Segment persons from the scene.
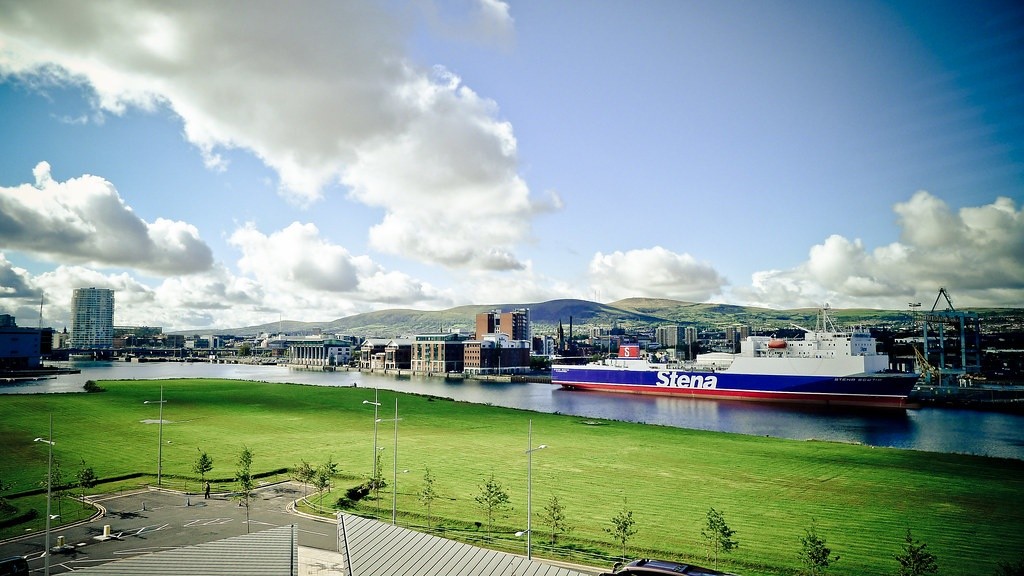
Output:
[711,362,716,373]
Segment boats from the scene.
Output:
[549,308,922,408]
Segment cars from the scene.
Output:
[596,558,737,576]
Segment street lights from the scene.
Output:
[362,400,385,490]
[375,417,409,526]
[143,400,171,485]
[514,444,549,560]
[33,437,60,576]
